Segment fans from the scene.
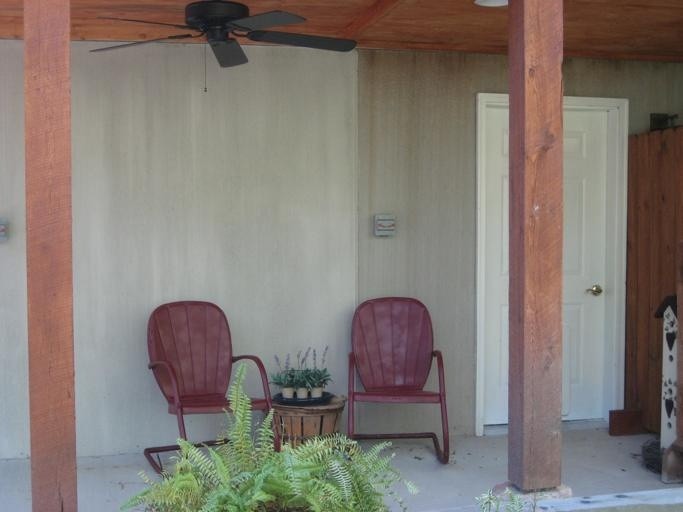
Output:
[87,0,358,70]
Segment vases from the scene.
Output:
[280,387,322,399]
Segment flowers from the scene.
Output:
[267,345,335,388]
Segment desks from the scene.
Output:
[270,392,346,464]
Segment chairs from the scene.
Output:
[141,299,282,486]
[346,295,449,466]
